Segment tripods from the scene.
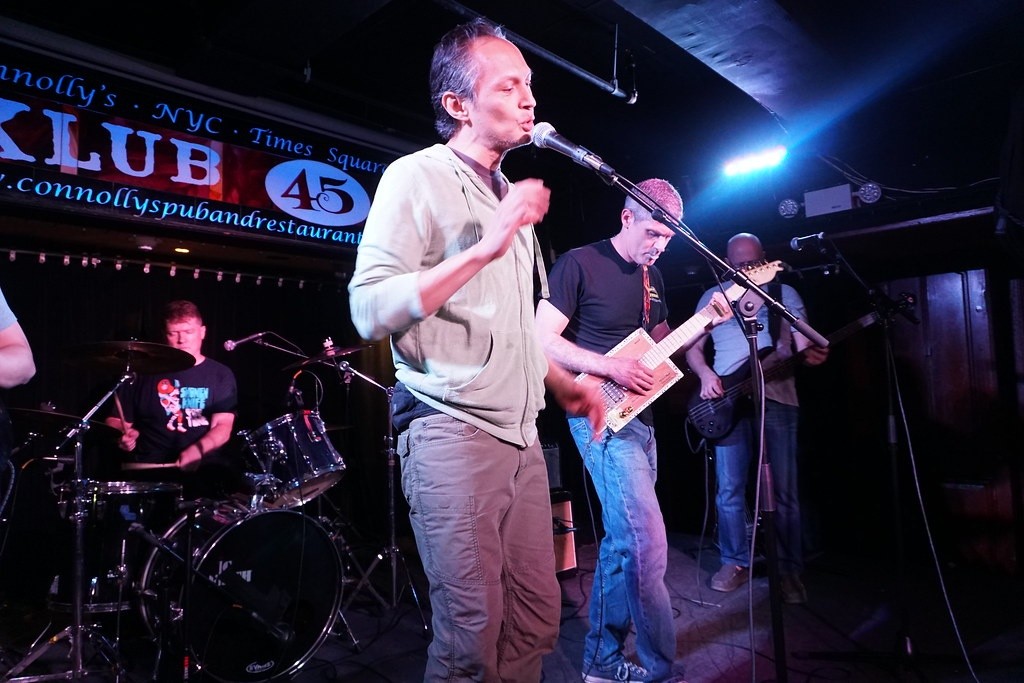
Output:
[0,358,139,683]
[318,362,432,642]
[792,245,986,683]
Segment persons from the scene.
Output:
[348,19,606,683]
[108,299,237,484]
[686,232,829,603]
[0,287,36,388]
[536,179,733,683]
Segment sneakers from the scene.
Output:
[711,564,750,592]
[583,662,648,683]
[781,578,807,604]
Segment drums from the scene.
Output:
[235,408,346,511]
[136,495,347,683]
[49,478,185,617]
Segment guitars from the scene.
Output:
[686,290,916,440]
[571,259,783,434]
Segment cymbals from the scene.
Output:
[5,404,125,443]
[73,339,198,382]
[279,343,375,375]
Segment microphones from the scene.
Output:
[284,379,296,407]
[274,621,296,647]
[532,121,616,177]
[223,332,266,351]
[552,528,576,534]
[790,232,825,250]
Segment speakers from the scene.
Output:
[551,489,579,579]
